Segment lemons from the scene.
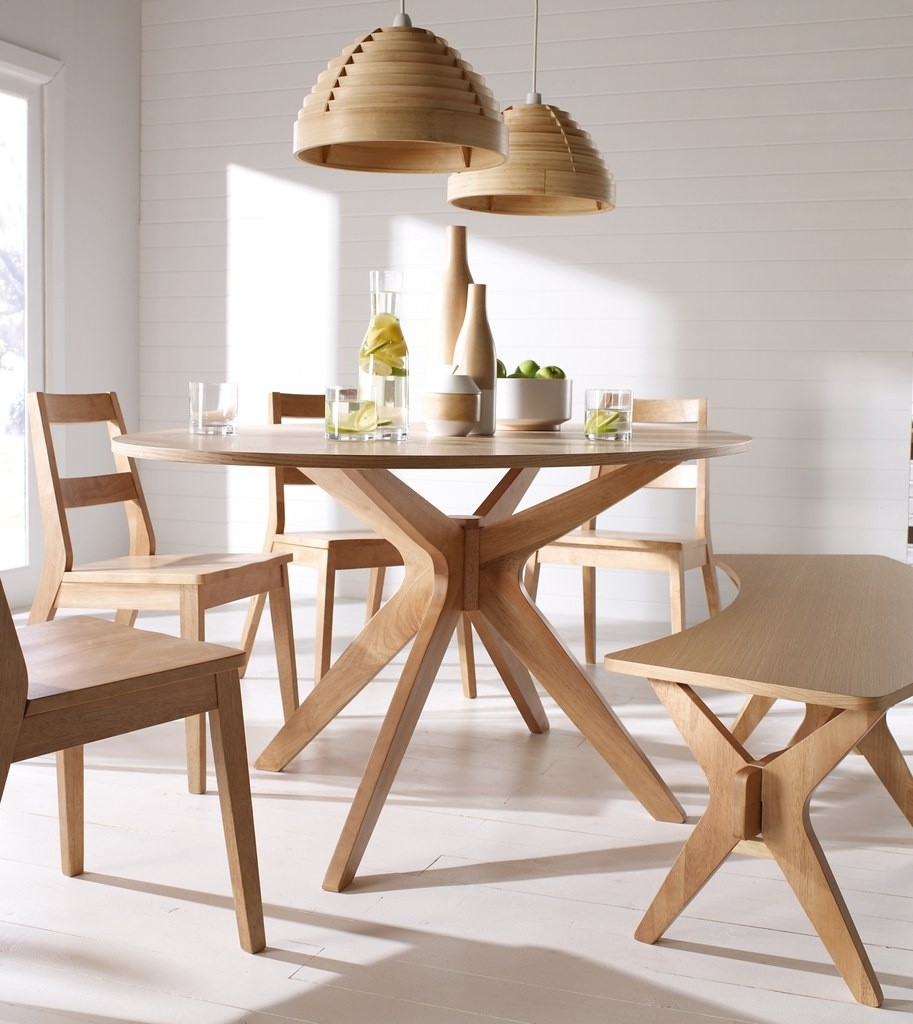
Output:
[584,409,621,433]
[326,402,391,433]
[359,312,407,377]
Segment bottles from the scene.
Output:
[359,270,410,442]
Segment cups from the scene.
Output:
[188,381,238,436]
[584,388,634,443]
[324,385,377,443]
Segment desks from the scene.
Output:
[118,424,756,895]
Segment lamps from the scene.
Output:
[447,0,617,218]
[293,0,510,173]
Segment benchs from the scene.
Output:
[606,553,913,1009]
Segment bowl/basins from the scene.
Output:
[496,377,573,431]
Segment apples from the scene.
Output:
[496,358,566,378]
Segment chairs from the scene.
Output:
[0,576,268,958]
[524,397,721,669]
[238,393,478,701]
[27,390,302,794]
